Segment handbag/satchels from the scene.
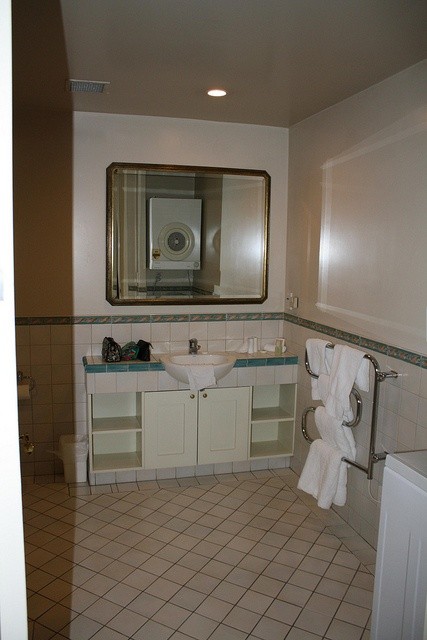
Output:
[102,337,122,362]
[122,341,140,360]
[137,339,153,361]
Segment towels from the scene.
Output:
[317,374,354,425]
[184,364,216,393]
[314,406,357,468]
[333,344,370,401]
[305,338,333,402]
[297,438,347,510]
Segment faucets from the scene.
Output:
[188,337,202,353]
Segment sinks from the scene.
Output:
[161,355,238,384]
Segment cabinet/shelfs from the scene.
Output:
[370,449,426,639]
[250,383,298,472]
[88,392,144,485]
[144,387,250,481]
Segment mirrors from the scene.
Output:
[106,162,271,306]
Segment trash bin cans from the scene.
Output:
[58,433,88,483]
